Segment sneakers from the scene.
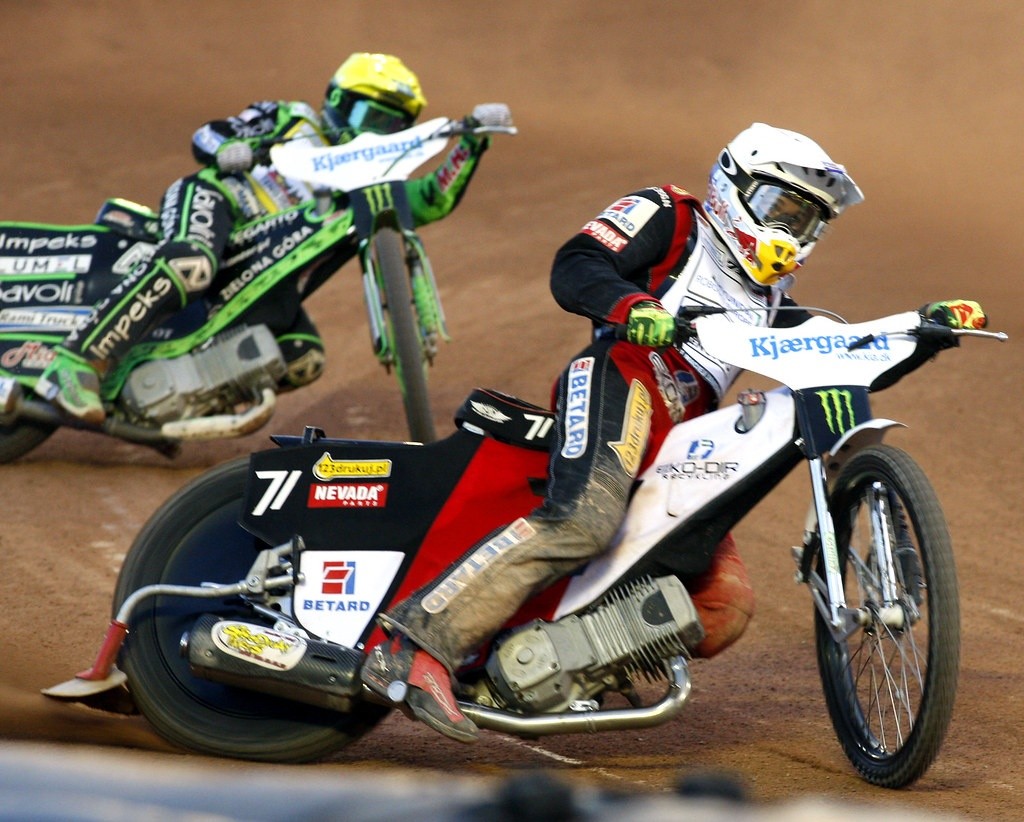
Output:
[34,353,106,425]
[361,631,481,745]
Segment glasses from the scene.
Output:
[325,84,409,135]
[717,147,831,245]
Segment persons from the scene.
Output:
[34,52,514,421]
[359,123,985,745]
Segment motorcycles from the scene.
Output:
[38,304,1012,792]
[0,102,524,469]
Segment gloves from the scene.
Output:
[626,299,679,347]
[467,102,513,138]
[918,298,986,330]
[215,137,254,175]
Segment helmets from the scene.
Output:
[702,122,865,287]
[320,52,430,146]
